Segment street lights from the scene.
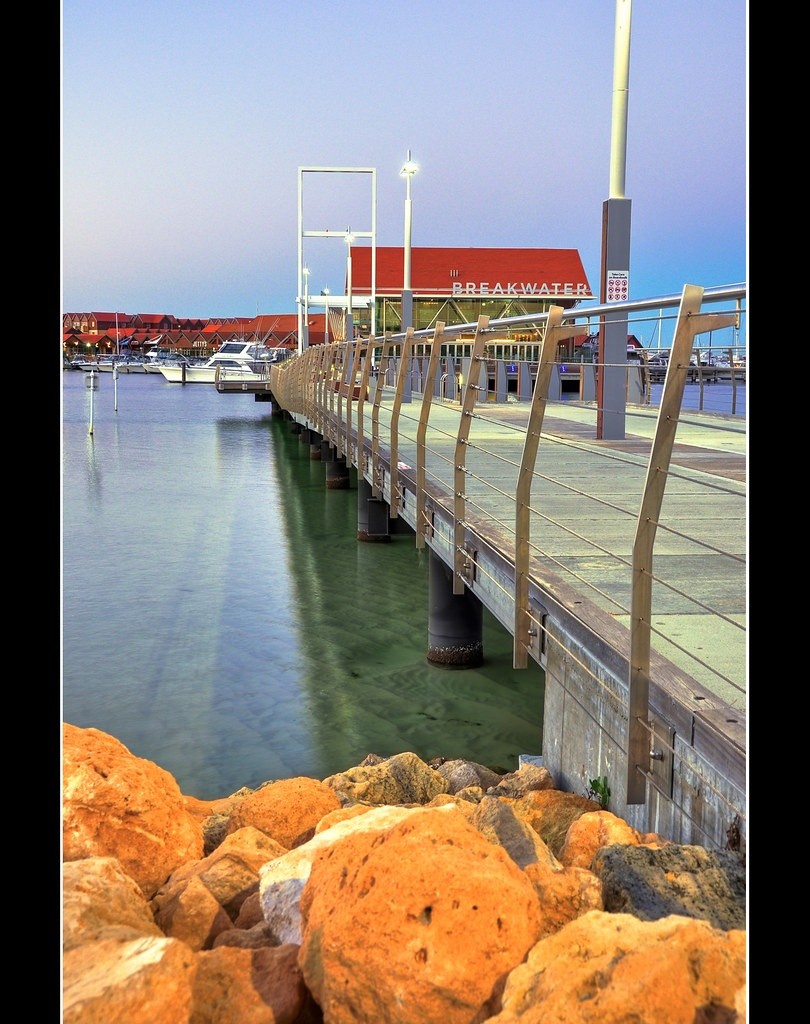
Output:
[344,225,354,384]
[324,283,330,372]
[302,262,310,351]
[399,149,419,403]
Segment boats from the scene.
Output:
[63,315,210,374]
[159,317,296,384]
[648,308,746,379]
